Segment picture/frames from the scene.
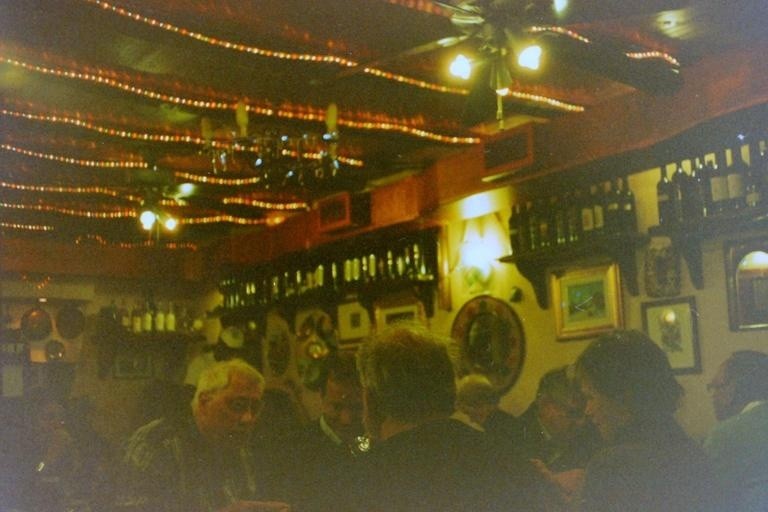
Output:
[641,295,704,377]
[113,353,153,379]
[547,259,624,343]
[335,291,427,351]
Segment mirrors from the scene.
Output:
[723,236,767,331]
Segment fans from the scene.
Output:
[326,0,687,82]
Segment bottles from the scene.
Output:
[330,243,431,287]
[654,130,768,221]
[222,272,263,313]
[507,171,636,253]
[264,253,329,300]
[97,286,207,336]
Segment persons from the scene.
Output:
[1,325,768,510]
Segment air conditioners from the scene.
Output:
[476,122,554,184]
[316,191,369,232]
